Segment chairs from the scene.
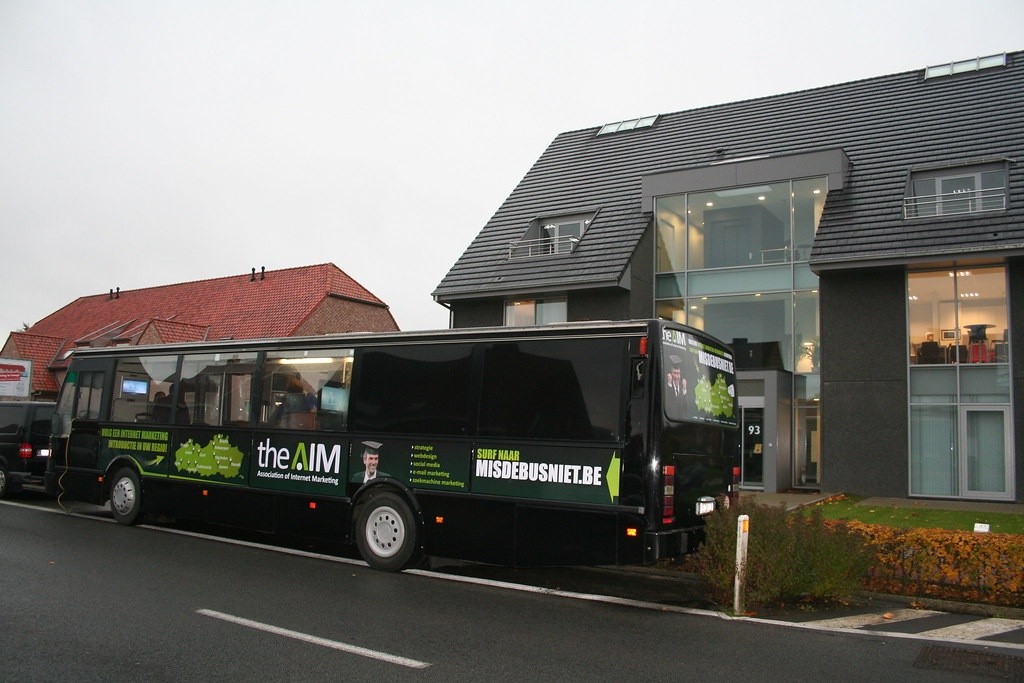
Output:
[951,344,967,363]
[277,412,317,431]
[921,342,940,364]
[989,329,1009,363]
[995,343,1008,363]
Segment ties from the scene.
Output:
[676,386,679,397]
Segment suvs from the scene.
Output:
[0,400,56,500]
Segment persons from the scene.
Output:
[666,355,690,419]
[267,383,308,425]
[146,383,191,425]
[352,440,392,483]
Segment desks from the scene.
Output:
[797,244,811,261]
[760,248,797,264]
[963,324,997,362]
[915,345,947,364]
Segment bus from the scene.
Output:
[45,317,738,573]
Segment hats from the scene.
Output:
[669,355,683,369]
[361,441,383,455]
